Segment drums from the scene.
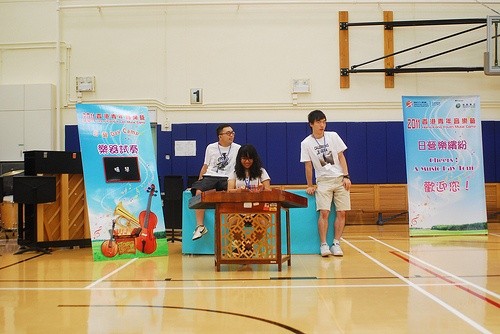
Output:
[2,194,26,230]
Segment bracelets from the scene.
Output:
[343,174,351,179]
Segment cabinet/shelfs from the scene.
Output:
[0,84,57,203]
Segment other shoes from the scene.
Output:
[232,246,252,258]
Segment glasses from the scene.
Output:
[219,131,235,136]
[241,157,254,162]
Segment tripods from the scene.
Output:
[12,175,57,255]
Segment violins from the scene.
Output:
[135,183,158,254]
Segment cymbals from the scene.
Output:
[0,169,24,178]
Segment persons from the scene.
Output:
[191,124,243,241]
[227,143,271,258]
[300,110,352,257]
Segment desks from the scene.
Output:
[182,188,336,253]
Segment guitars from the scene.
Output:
[101,219,118,257]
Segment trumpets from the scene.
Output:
[113,201,141,229]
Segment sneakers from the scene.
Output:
[330,243,343,256]
[192,224,208,240]
[320,242,332,256]
